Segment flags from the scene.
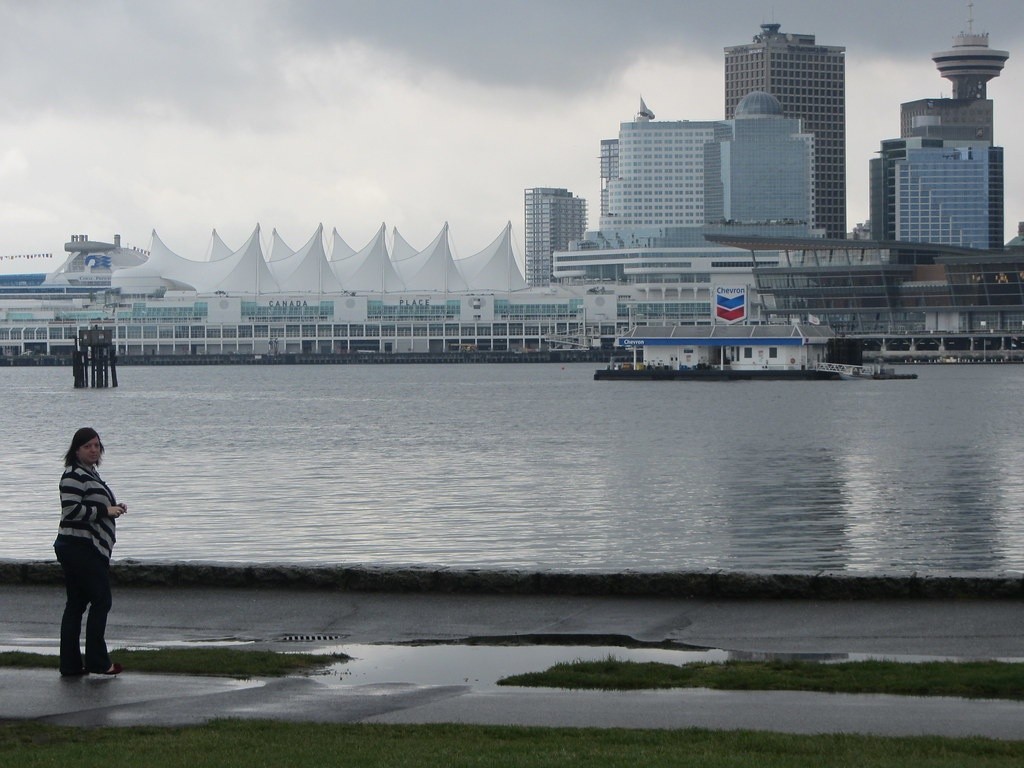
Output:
[809,315,820,325]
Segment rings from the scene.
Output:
[117,511,120,513]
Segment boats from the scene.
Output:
[0,233,195,320]
[594,325,919,380]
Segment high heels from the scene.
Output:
[58,664,88,676]
[106,662,124,675]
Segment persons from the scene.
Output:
[53,427,128,676]
